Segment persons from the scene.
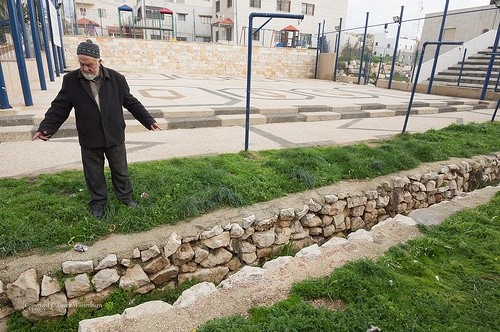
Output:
[32,39,162,220]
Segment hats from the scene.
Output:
[76,39,101,58]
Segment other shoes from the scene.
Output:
[119,195,138,210]
[90,206,108,221]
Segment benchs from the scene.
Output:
[427,46,500,91]
